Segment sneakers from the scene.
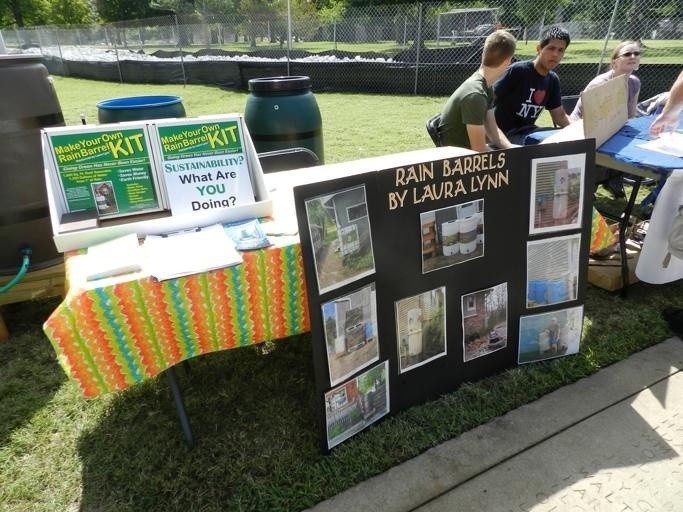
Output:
[602,182,626,198]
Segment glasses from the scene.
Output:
[613,51,640,58]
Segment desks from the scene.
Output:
[63,145,488,449]
[524,107,683,300]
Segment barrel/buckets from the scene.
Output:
[0,53,66,276]
[244,74,323,167]
[95,95,187,124]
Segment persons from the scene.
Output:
[569,37,642,135]
[649,70,683,343]
[426,30,516,155]
[494,24,570,146]
